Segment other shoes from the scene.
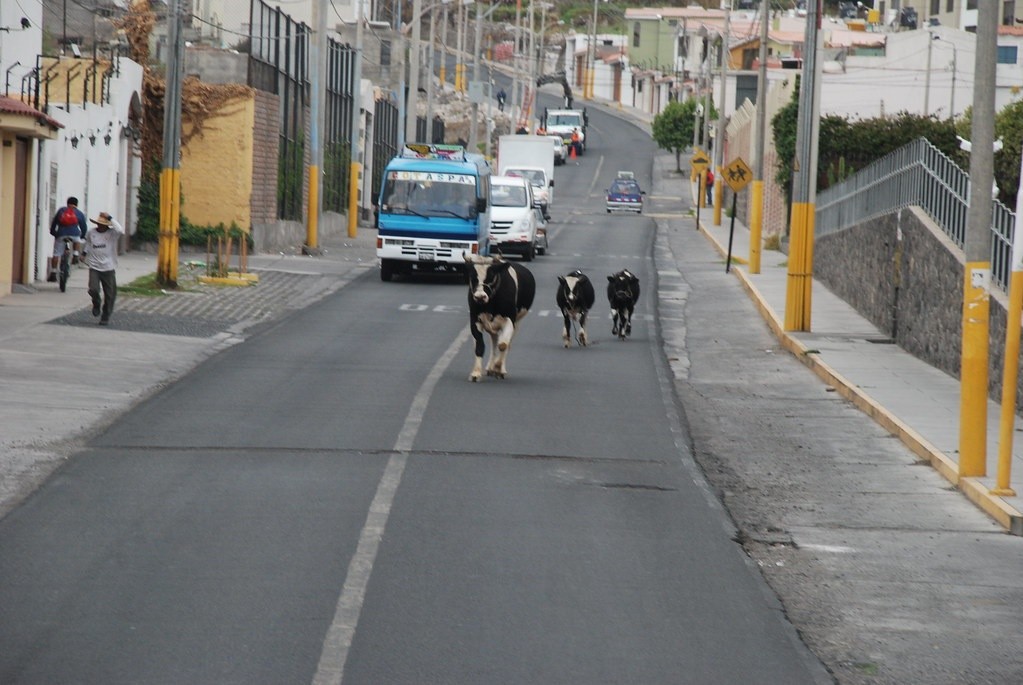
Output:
[72,255,78,264]
[99,318,110,326]
[50,272,56,282]
[92,305,101,317]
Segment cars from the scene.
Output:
[604,180,646,214]
[534,206,552,252]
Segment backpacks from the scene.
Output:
[60,206,78,226]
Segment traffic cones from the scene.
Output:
[570,147,577,159]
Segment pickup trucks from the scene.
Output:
[547,135,569,164]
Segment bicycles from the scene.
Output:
[57,238,73,291]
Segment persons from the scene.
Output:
[571,128,580,154]
[443,188,469,207]
[80,212,125,325]
[50,197,87,293]
[497,88,506,111]
[706,169,713,205]
[536,127,546,135]
[519,124,528,134]
[388,186,411,211]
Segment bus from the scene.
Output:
[376,142,493,282]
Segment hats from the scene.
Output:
[90,213,112,225]
[68,197,78,206]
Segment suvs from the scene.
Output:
[490,176,541,260]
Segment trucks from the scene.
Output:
[545,108,587,149]
[497,133,555,213]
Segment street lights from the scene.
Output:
[655,14,687,104]
[506,19,564,118]
[424,1,475,146]
[933,35,956,122]
[397,0,450,158]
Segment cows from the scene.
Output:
[461,249,535,382]
[555,269,595,349]
[606,269,641,341]
